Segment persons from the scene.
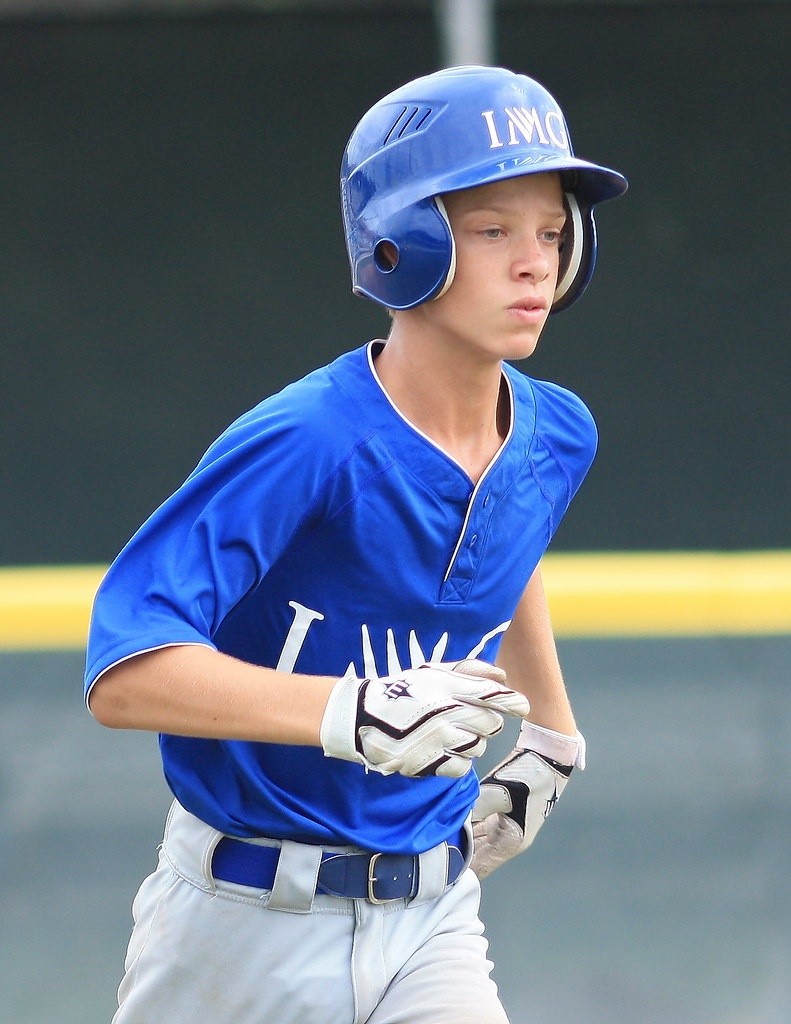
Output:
[87,66,626,1024]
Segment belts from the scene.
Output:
[212,832,470,907]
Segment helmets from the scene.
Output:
[338,62,626,312]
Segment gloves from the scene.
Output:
[321,658,529,779]
[470,722,587,879]
